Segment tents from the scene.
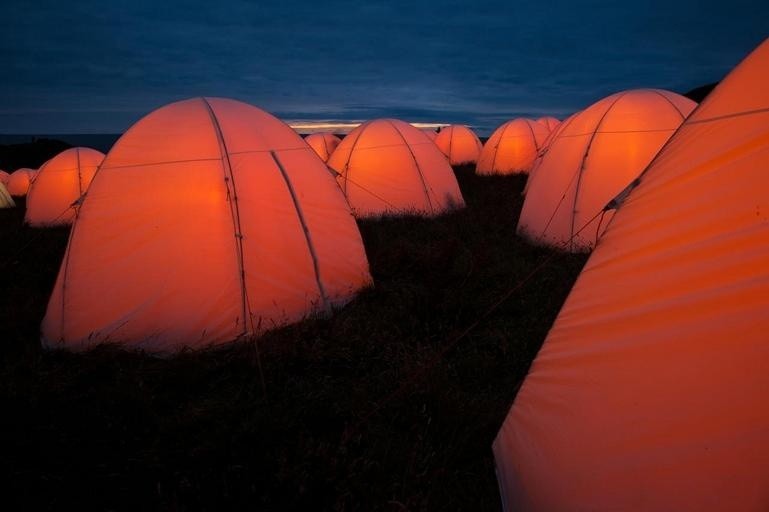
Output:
[521,111,582,195]
[0,170,10,185]
[424,129,439,141]
[6,168,39,196]
[435,124,483,166]
[0,182,16,208]
[492,36,769,512]
[39,96,375,354]
[326,118,467,220]
[304,132,342,163]
[515,87,699,254]
[535,116,561,131]
[475,118,550,176]
[25,146,107,227]
